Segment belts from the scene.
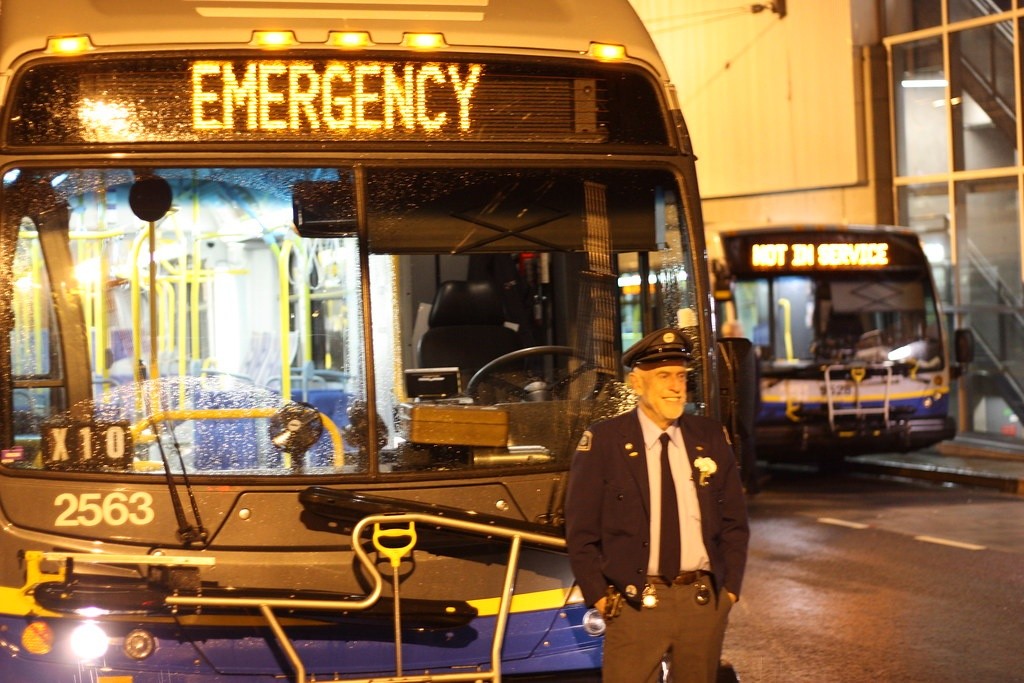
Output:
[644,569,710,585]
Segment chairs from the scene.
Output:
[13,328,357,470]
[414,279,542,384]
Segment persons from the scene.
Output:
[720,321,768,494]
[565,328,751,683]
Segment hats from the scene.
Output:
[620,328,693,371]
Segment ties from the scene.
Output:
[658,432,681,586]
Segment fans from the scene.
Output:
[269,402,324,474]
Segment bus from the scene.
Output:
[613,223,976,453]
[2,1,761,683]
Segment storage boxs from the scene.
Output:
[396,396,509,450]
